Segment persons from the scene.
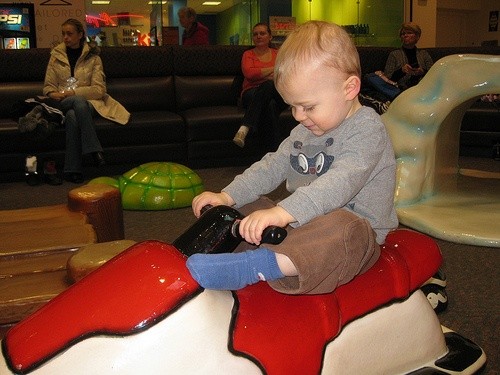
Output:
[179,7,209,48]
[384,23,433,90]
[185,20,398,294]
[43,18,131,184]
[233,23,287,155]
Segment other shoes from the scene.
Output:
[233,135,244,148]
[65,174,84,183]
[94,151,106,167]
[25,172,39,186]
[44,173,62,185]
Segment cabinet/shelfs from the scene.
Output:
[100,26,123,48]
[120,24,144,47]
[0,2,37,50]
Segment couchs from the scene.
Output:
[0,46,500,181]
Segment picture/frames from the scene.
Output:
[150,25,158,46]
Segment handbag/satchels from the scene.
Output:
[19,105,52,132]
